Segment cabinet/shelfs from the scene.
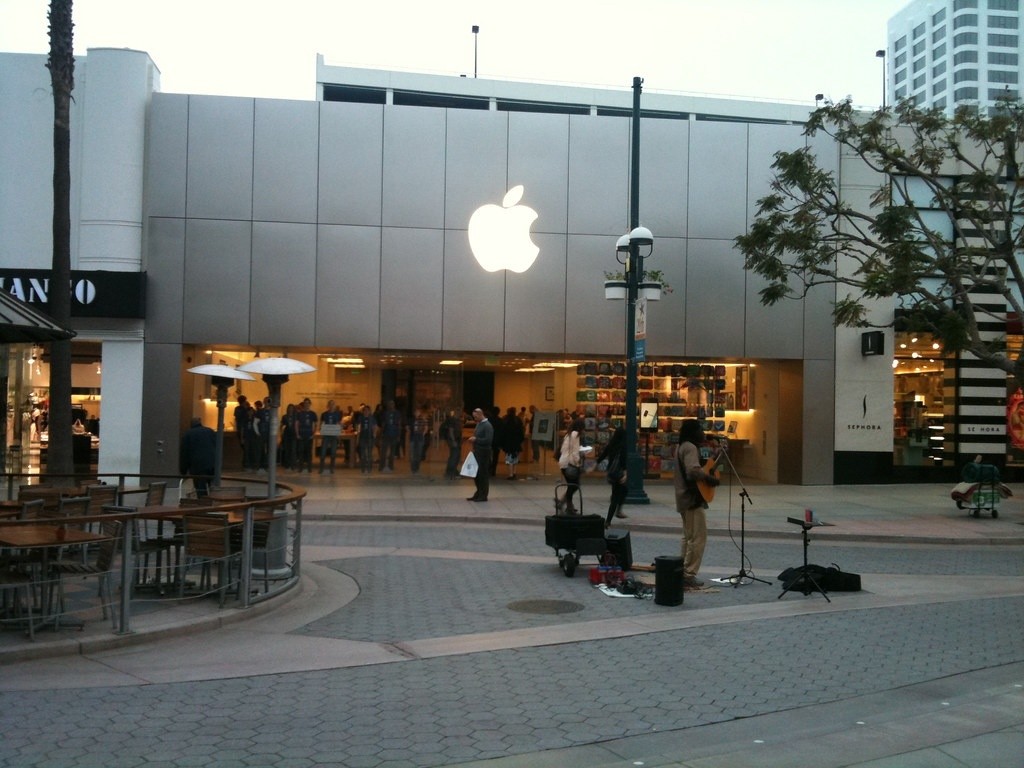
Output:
[728,439,750,464]
[893,401,936,467]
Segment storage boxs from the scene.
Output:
[735,366,756,411]
[961,490,1001,509]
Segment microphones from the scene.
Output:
[706,434,727,442]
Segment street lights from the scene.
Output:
[605,77,661,505]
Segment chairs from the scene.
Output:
[0,479,286,644]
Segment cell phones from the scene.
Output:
[639,398,658,434]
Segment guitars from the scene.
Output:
[696,444,728,503]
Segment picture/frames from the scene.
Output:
[546,387,554,401]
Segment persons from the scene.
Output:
[463,407,493,501]
[179,417,217,498]
[554,421,585,514]
[88,414,97,424]
[663,416,674,433]
[586,425,628,532]
[232,394,271,471]
[444,406,463,478]
[673,420,721,586]
[279,397,407,475]
[410,402,434,476]
[490,402,542,479]
[556,408,578,428]
[41,408,48,430]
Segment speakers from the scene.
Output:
[602,528,638,569]
[654,555,685,606]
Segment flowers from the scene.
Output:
[602,268,626,281]
[644,270,674,297]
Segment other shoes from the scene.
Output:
[467,494,487,502]
[683,572,704,588]
[616,512,628,518]
[558,502,577,511]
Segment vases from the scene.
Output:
[641,279,662,301]
[603,280,625,300]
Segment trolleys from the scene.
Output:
[956,455,998,519]
[554,484,618,577]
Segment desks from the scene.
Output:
[312,431,360,470]
[22,487,151,555]
[0,524,107,636]
[133,506,281,598]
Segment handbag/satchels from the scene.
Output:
[566,464,581,479]
[609,442,630,483]
[460,449,477,477]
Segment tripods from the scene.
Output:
[716,439,775,588]
[778,514,833,603]
